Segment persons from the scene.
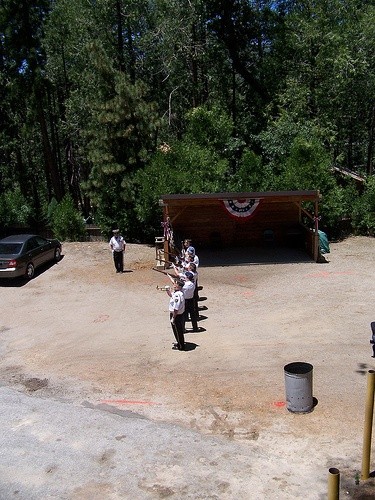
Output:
[165,239,200,350]
[109,228,127,274]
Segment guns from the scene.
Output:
[156,258,183,268]
[153,268,179,278]
[161,250,184,260]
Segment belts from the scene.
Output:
[114,250,123,253]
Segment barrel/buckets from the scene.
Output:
[283,362,314,412]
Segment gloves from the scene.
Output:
[175,255,180,263]
[170,316,175,326]
[165,285,170,289]
[170,262,177,270]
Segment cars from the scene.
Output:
[0,234,63,280]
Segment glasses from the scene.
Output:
[113,233,119,234]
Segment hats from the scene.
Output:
[189,262,196,271]
[185,271,192,280]
[187,246,196,254]
[112,229,120,232]
[184,239,193,244]
[174,278,185,287]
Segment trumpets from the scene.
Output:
[155,284,172,291]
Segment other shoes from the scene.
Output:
[116,270,123,274]
[172,343,186,352]
[193,326,203,333]
[184,328,189,334]
